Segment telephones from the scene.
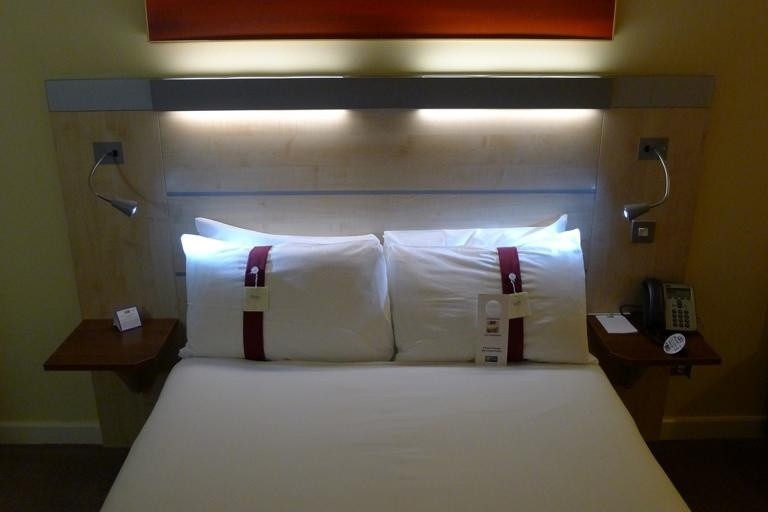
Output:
[640,276,698,334]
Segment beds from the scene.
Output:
[41,71,721,511]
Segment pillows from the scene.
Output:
[180,214,599,365]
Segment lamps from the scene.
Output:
[86,140,141,218]
[622,135,673,223]
